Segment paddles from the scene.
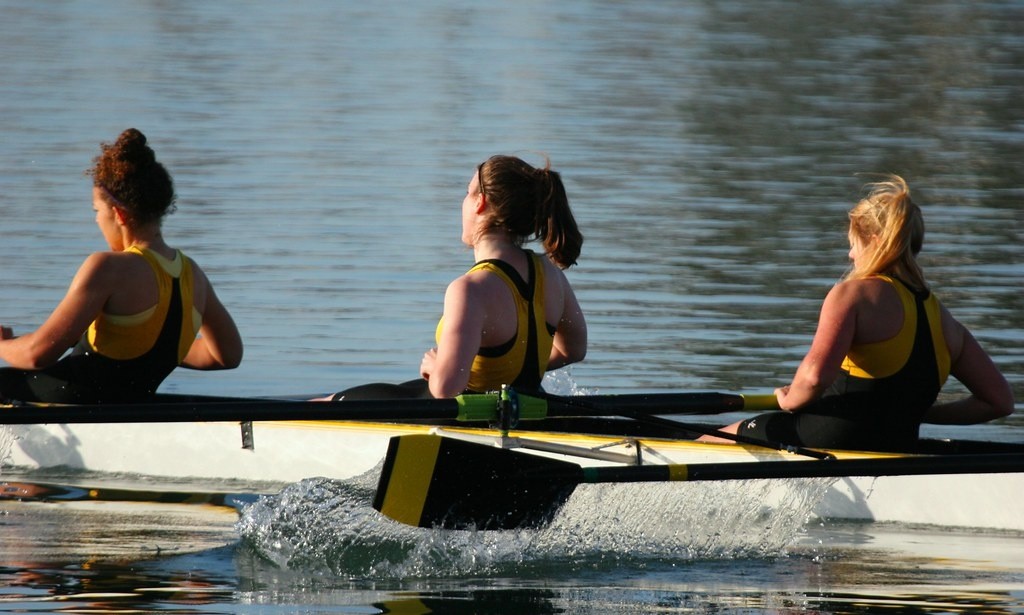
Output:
[0,393,784,424]
[373,433,1024,532]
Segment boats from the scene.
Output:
[0,363,1024,535]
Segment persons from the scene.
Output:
[0,125,244,412]
[691,172,1018,455]
[301,152,588,410]
